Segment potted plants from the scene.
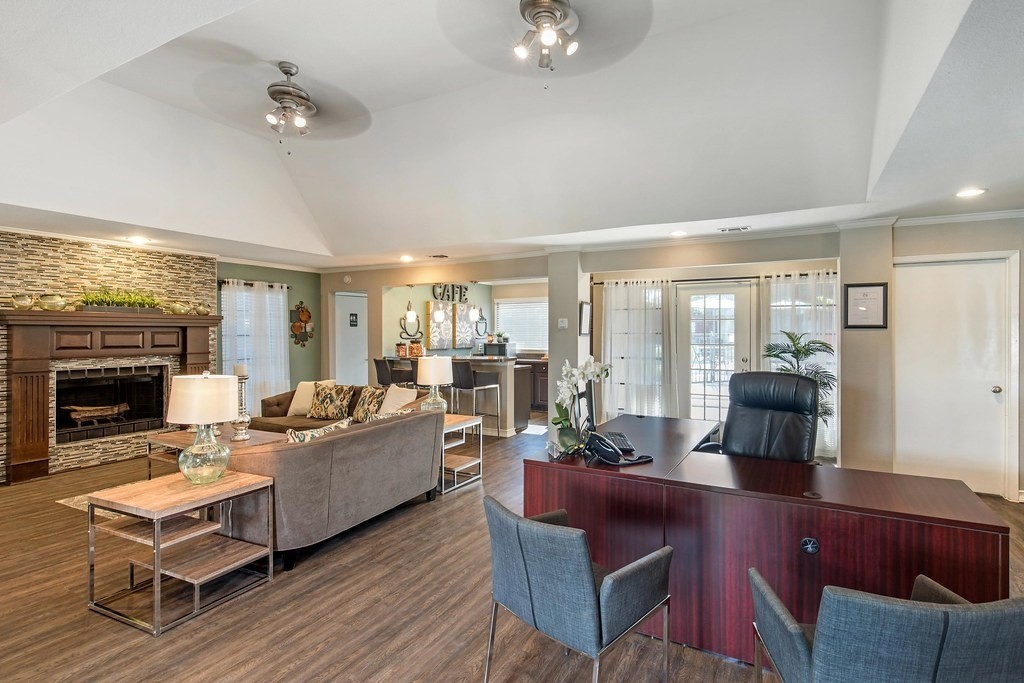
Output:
[74,286,164,313]
[496,330,505,343]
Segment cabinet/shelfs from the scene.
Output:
[531,364,547,412]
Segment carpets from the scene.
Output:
[54,479,200,520]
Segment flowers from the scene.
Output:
[555,353,614,410]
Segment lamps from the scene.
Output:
[514,0,579,89]
[166,370,238,484]
[470,280,479,322]
[434,283,445,322]
[405,284,417,322]
[418,356,454,412]
[265,61,318,155]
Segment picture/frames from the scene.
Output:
[579,301,591,336]
[843,282,888,328]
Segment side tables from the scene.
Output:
[87,465,275,638]
[436,414,483,495]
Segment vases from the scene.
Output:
[558,386,592,457]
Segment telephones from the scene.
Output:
[588,432,623,463]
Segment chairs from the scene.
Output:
[747,566,1024,683]
[698,371,823,466]
[372,357,501,444]
[484,495,673,683]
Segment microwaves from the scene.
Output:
[484,342,516,357]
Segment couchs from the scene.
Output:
[209,410,446,570]
[248,386,442,434]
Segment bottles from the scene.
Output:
[409,340,423,357]
[395,343,407,357]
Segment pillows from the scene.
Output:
[353,384,386,423]
[365,408,416,422]
[285,416,353,443]
[287,379,336,415]
[307,381,355,419]
[376,384,418,414]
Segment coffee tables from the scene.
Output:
[147,425,287,481]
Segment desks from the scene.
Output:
[664,451,1010,674]
[523,414,720,640]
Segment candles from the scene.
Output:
[233,363,247,376]
